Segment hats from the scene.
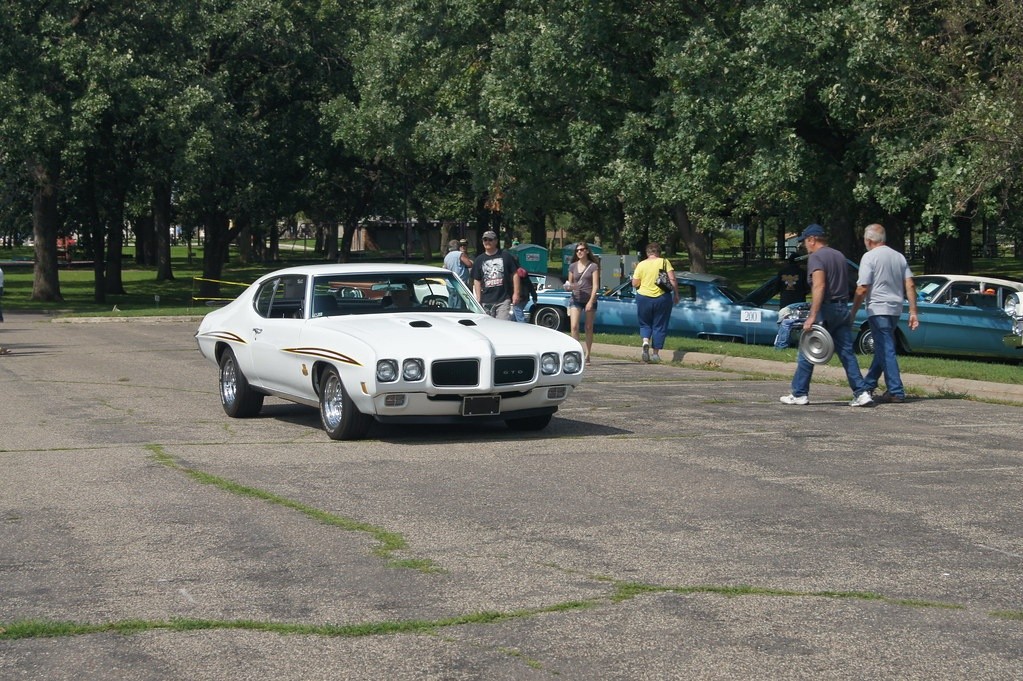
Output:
[459,239,469,245]
[482,231,497,240]
[787,253,803,266]
[795,223,825,242]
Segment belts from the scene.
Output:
[829,299,847,304]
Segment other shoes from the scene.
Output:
[641,342,650,362]
[876,390,905,403]
[650,354,662,364]
[584,359,591,366]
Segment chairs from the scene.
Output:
[380,296,393,308]
[315,295,339,317]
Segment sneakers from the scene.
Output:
[779,393,810,405]
[849,391,874,407]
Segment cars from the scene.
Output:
[523,270,780,345]
[776,249,1023,365]
[326,267,563,321]
[193,263,586,441]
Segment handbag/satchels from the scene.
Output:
[566,300,571,317]
[654,258,673,293]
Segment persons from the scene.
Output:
[401,241,405,255]
[631,241,679,363]
[849,224,919,404]
[511,236,519,247]
[508,255,537,323]
[470,231,520,320]
[616,239,625,254]
[562,241,600,366]
[443,238,473,311]
[0,267,11,356]
[779,224,875,409]
[776,253,811,308]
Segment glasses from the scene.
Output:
[483,238,494,242]
[575,247,588,253]
